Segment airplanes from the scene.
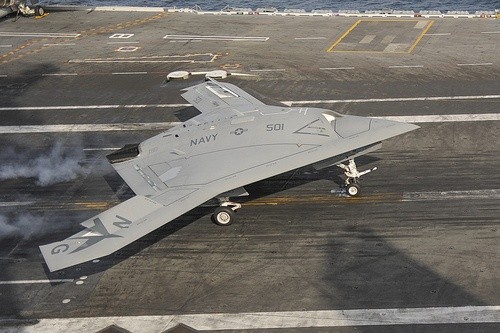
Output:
[37,78,423,273]
[0,0,83,20]
